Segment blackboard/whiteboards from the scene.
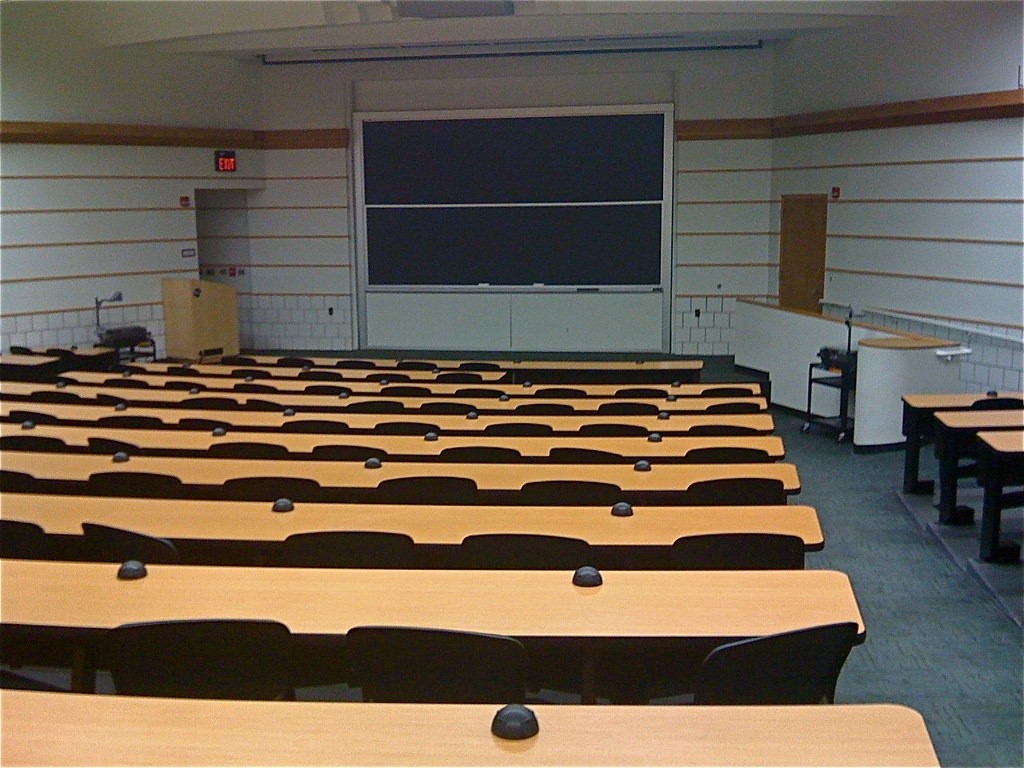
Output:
[353,104,672,294]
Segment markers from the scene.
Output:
[577,288,600,291]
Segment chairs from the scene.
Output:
[1,346,1024,704]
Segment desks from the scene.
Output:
[0,354,1024,768]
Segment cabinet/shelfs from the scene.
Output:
[92,339,155,362]
[807,364,854,444]
